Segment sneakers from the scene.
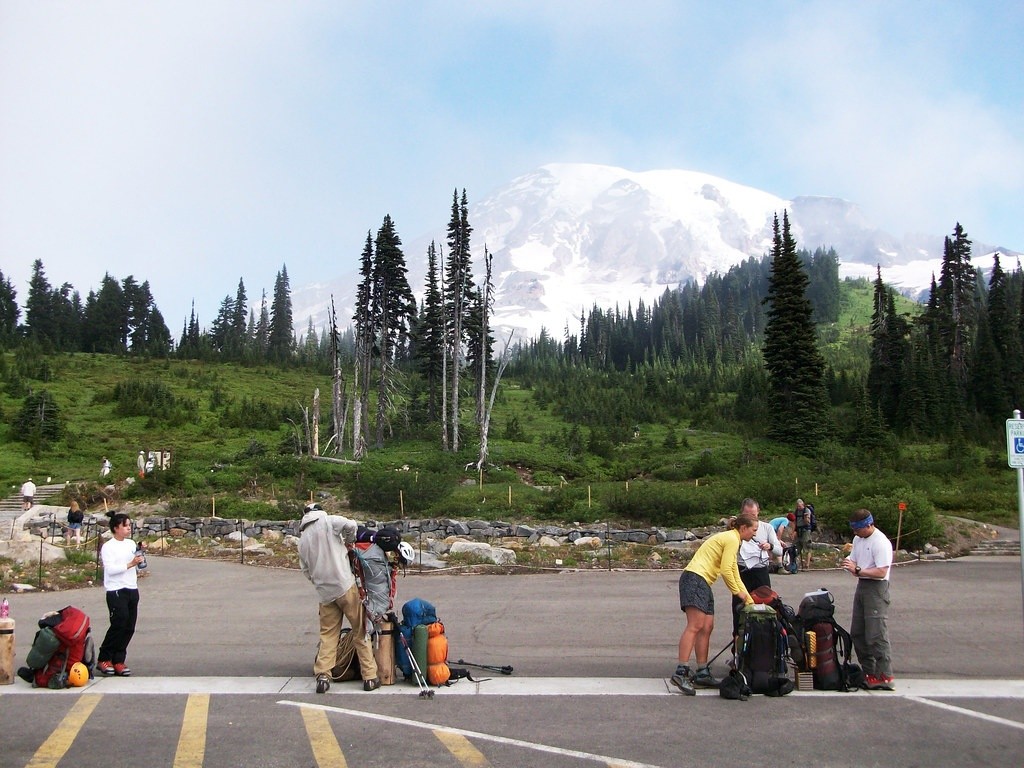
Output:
[693,675,721,689]
[364,678,380,691]
[779,567,791,575]
[670,673,696,696]
[316,675,330,693]
[864,672,895,689]
[96,660,115,675]
[802,567,810,572]
[113,662,130,676]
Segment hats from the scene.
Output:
[787,513,796,522]
[28,478,33,480]
[140,451,145,454]
[303,503,323,514]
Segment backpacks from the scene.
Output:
[346,541,393,622]
[782,547,798,573]
[391,598,493,688]
[17,606,95,690]
[720,588,865,700]
[795,504,817,532]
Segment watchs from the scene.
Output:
[855,566,861,574]
[769,543,774,551]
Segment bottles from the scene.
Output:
[0,597,9,618]
[133,546,147,569]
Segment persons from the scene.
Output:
[97,510,144,676]
[767,513,796,574]
[794,499,812,571]
[732,496,784,656]
[138,450,145,477]
[99,457,111,476]
[66,501,84,550]
[840,509,895,691]
[298,503,381,693]
[21,478,36,511]
[670,514,756,695]
